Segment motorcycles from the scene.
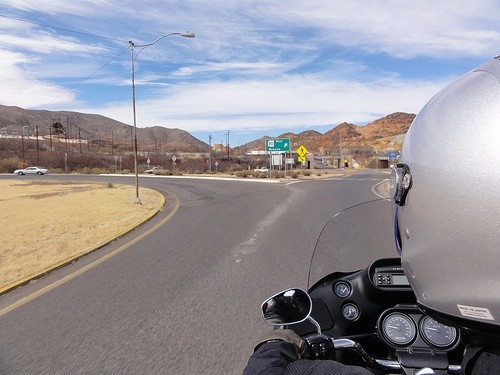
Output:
[260,196,474,375]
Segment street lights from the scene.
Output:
[128,31,195,194]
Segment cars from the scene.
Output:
[14,167,48,175]
[144,166,169,175]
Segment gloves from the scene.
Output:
[251,328,308,360]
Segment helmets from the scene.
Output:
[393,55,500,328]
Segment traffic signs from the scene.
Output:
[264,138,290,152]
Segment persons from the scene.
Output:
[240,54,500,375]
